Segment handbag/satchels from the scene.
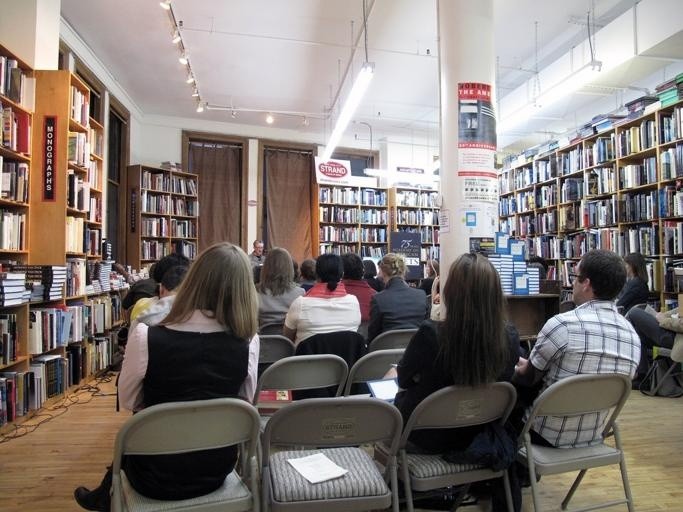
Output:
[640,353,682,398]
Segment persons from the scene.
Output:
[628,306,683,391]
[247,239,442,382]
[117,252,191,359]
[75,241,262,512]
[471,249,642,497]
[380,254,522,511]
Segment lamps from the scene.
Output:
[206,106,322,125]
[496,0,603,130]
[157,1,206,113]
[323,0,376,165]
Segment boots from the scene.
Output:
[75,465,113,511]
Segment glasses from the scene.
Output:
[567,271,582,282]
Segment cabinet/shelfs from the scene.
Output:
[320,183,440,280]
[0,44,199,438]
[489,100,683,305]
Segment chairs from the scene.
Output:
[112,398,262,512]
[223,327,421,480]
[241,397,404,511]
[503,372,634,511]
[374,381,516,510]
[639,348,683,397]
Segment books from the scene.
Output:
[0,55,68,307]
[487,232,539,296]
[1,293,122,425]
[64,257,124,298]
[500,72,683,311]
[141,170,196,261]
[67,85,103,256]
[318,187,440,279]
